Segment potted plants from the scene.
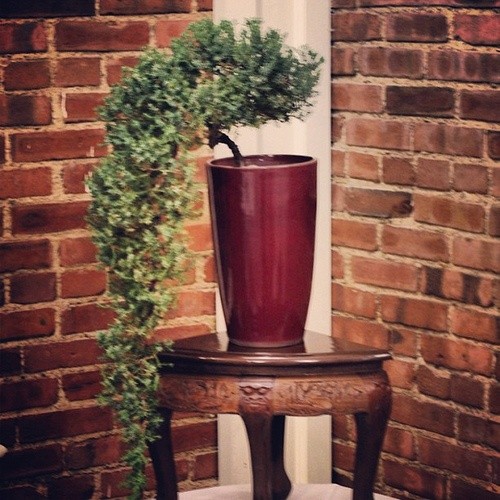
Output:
[82,14,326,499]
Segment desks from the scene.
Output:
[143,329,396,500]
